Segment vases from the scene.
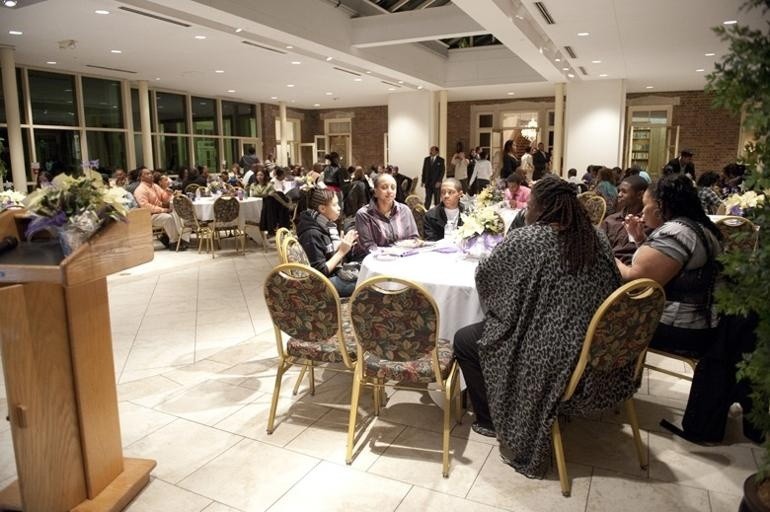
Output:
[58,223,99,261]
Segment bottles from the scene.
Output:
[238,188,248,201]
[444,220,453,245]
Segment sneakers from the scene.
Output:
[472,422,497,436]
[460,390,473,412]
[170,242,187,250]
[159,233,169,247]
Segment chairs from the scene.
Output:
[148,179,276,259]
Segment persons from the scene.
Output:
[422,141,550,211]
[567,149,747,359]
[34,148,413,251]
[297,172,468,296]
[453,177,646,480]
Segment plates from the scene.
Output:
[370,239,421,257]
[433,246,458,253]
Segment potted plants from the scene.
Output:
[705,1,770,506]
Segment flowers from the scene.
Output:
[0,160,140,244]
